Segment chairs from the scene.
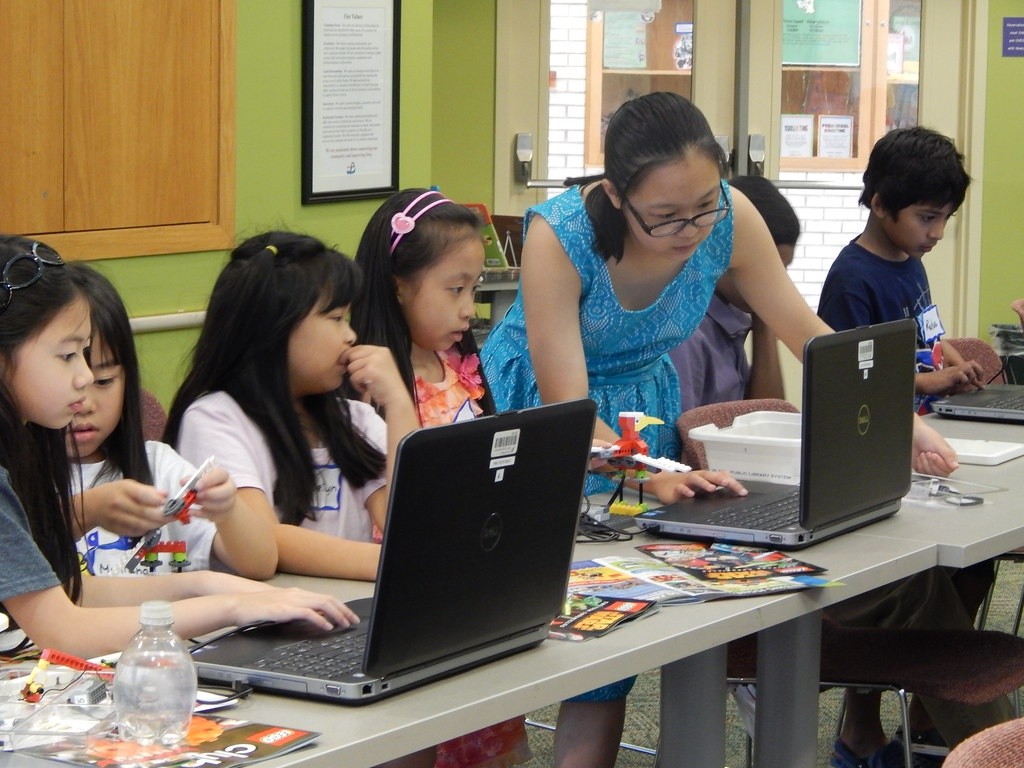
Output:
[142,297,1024,768]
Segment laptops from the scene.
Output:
[929,380,1024,420]
[634,318,918,549]
[189,399,598,704]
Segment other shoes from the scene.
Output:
[892,727,949,768]
[830,739,907,768]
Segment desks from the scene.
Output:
[0,518,938,765]
[750,409,1024,766]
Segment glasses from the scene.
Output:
[616,179,732,238]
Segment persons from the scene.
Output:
[162,229,426,766]
[2,235,361,664]
[481,93,959,767]
[354,186,538,768]
[816,124,994,768]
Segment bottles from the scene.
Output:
[112,600,198,747]
